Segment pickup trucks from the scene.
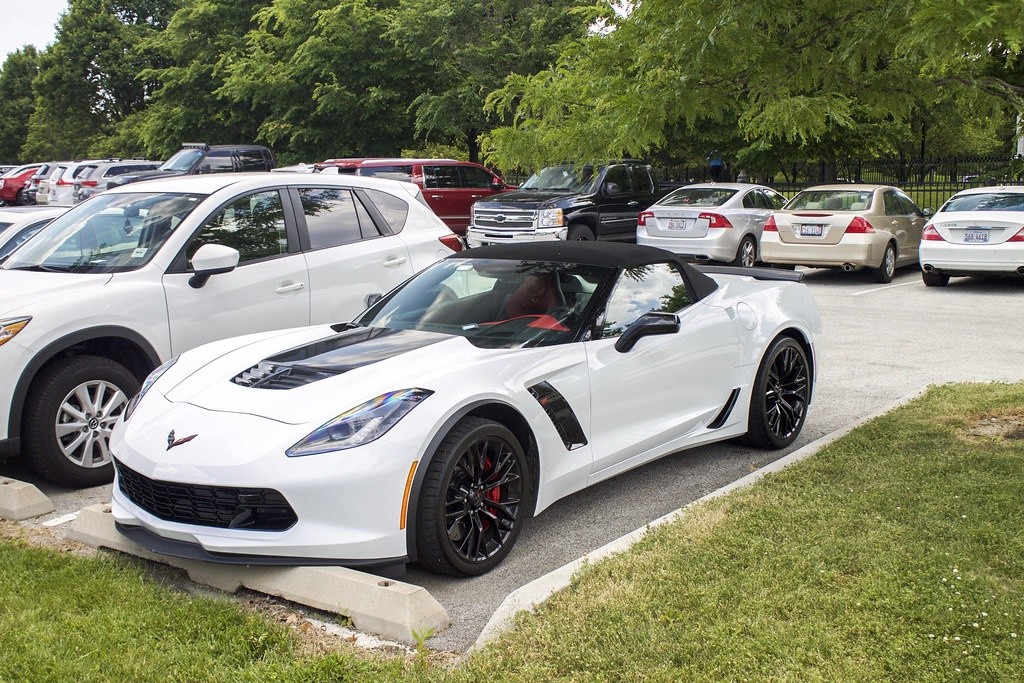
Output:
[466,157,686,250]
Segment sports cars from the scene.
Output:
[107,238,826,580]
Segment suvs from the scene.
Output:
[0,145,521,487]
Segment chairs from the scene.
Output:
[850,202,866,212]
[825,197,841,210]
[806,202,824,209]
[497,269,565,327]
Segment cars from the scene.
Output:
[961,174,997,185]
[636,181,789,268]
[919,185,1024,288]
[759,184,934,284]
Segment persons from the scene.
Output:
[707,150,726,181]
